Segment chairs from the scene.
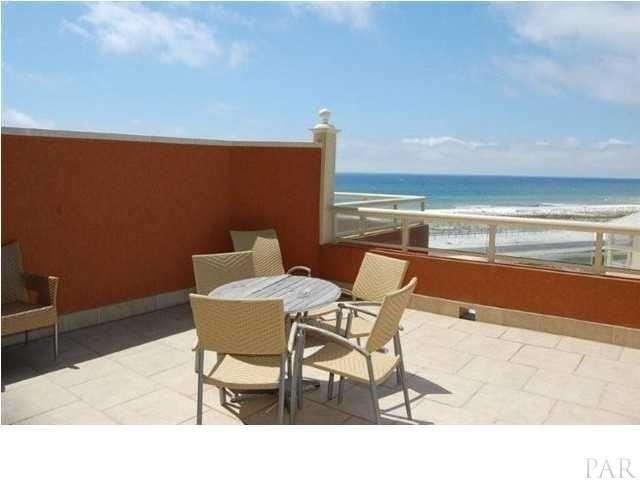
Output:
[186,228,419,424]
[2,241,60,363]
